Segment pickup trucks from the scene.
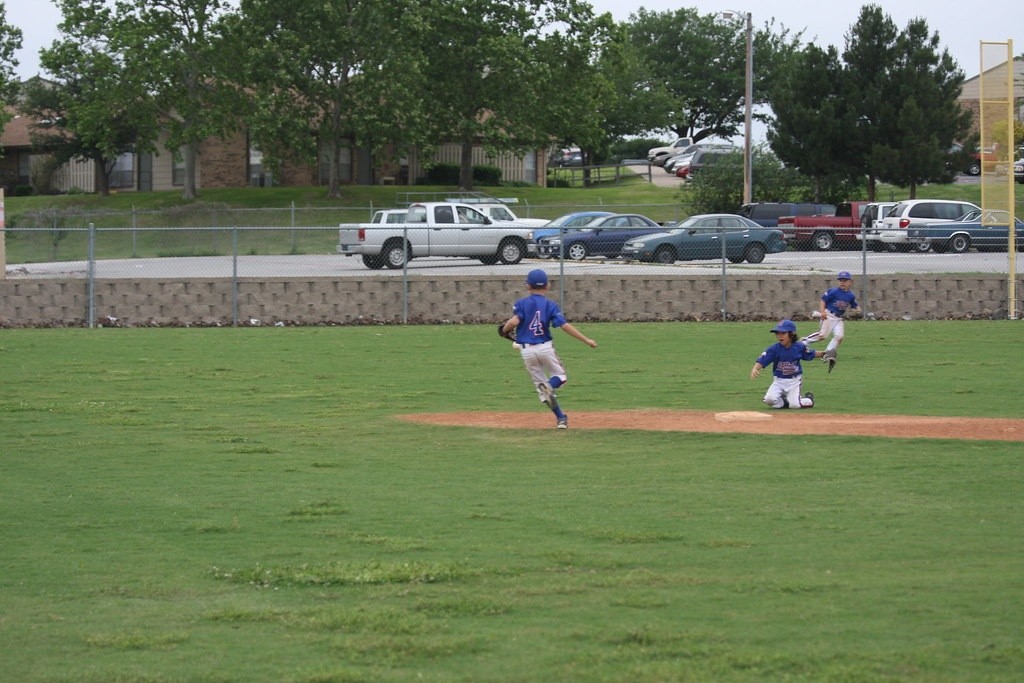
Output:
[647,137,693,162]
[338,202,537,269]
[778,201,873,251]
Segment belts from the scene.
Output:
[522,343,539,349]
[831,311,843,318]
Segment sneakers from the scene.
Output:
[557,414,568,428]
[538,382,556,407]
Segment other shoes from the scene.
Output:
[805,391,814,406]
[781,394,789,408]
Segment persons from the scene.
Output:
[796,271,862,362]
[498,270,599,429]
[750,319,837,409]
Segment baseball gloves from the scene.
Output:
[497,324,516,341]
[840,309,864,321]
[821,349,837,373]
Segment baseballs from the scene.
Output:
[512,342,521,350]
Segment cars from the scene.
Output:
[539,212,669,261]
[854,202,897,252]
[909,210,1024,252]
[370,208,482,224]
[469,203,551,259]
[620,213,788,264]
[880,200,1005,253]
[740,201,838,251]
[654,144,743,167]
[670,148,741,182]
[551,148,587,169]
[529,212,628,260]
[946,140,1024,184]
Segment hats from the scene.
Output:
[526,269,547,288]
[837,271,851,280]
[770,320,796,333]
